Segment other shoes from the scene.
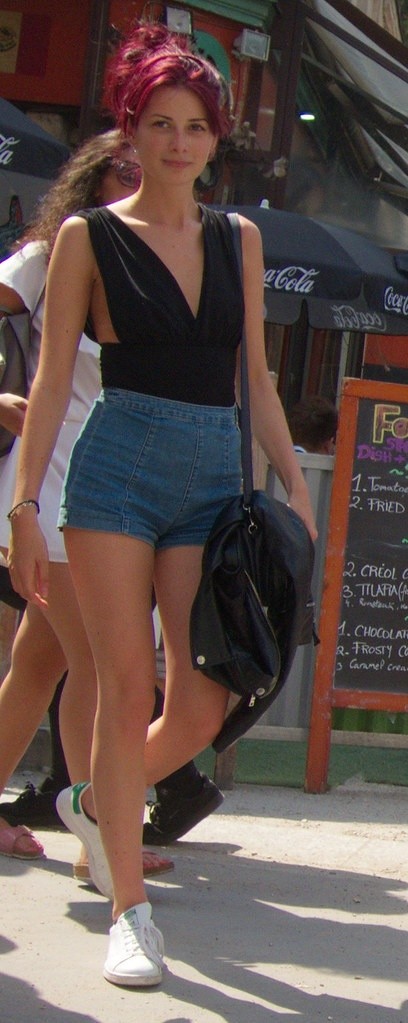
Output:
[1,777,70,824]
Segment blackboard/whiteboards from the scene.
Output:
[310,377,408,714]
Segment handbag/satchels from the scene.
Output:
[0,307,34,457]
[192,489,320,754]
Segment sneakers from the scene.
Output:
[142,774,225,846]
[103,900,165,987]
[56,781,115,899]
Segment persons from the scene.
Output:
[286,395,339,458]
[7,20,319,986]
[0,130,231,885]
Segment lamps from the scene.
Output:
[231,29,272,62]
[157,7,193,37]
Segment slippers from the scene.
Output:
[73,846,176,884]
[1,824,45,859]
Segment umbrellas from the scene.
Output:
[200,203,407,335]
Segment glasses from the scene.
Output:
[114,158,141,189]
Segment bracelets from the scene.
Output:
[6,499,39,521]
